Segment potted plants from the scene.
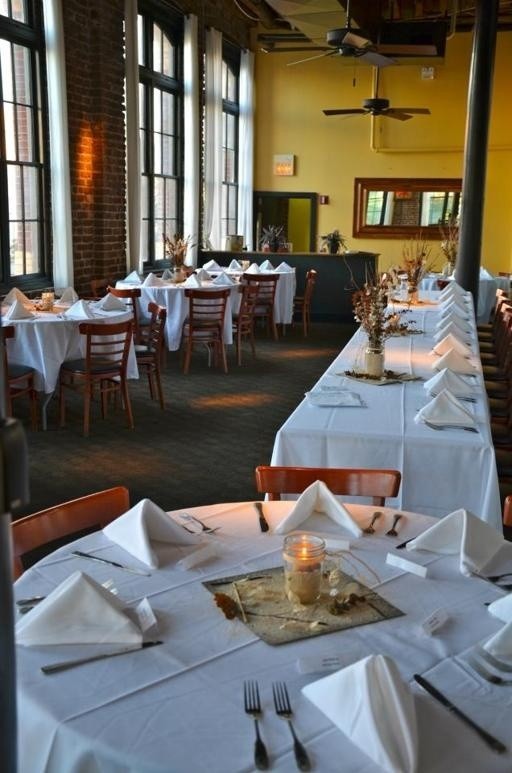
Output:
[319,229,349,254]
[163,234,195,282]
[257,223,285,252]
[439,216,459,276]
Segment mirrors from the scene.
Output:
[352,177,463,240]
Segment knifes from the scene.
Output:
[395,536,416,549]
[40,641,163,674]
[413,673,506,754]
[71,551,151,577]
[254,503,269,532]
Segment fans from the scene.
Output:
[261,0,398,70]
[322,64,432,122]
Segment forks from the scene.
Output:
[271,680,312,772]
[16,578,114,606]
[386,514,402,537]
[243,679,269,771]
[20,587,120,615]
[361,512,381,534]
[180,512,222,533]
[469,569,512,583]
